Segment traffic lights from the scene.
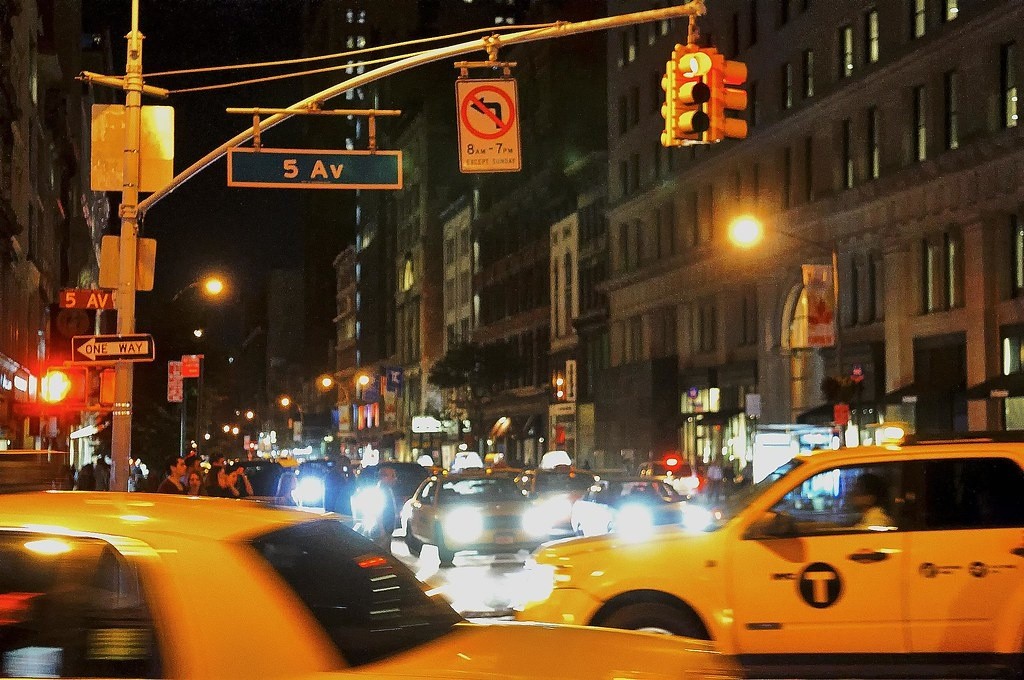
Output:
[717,57,748,139]
[675,51,714,137]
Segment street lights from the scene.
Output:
[189,367,369,454]
[157,270,228,349]
[724,213,849,447]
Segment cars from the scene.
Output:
[569,474,713,536]
[400,466,552,568]
[518,467,650,530]
[511,437,1024,679]
[263,453,432,516]
[0,489,745,679]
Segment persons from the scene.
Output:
[695,457,734,497]
[778,470,894,533]
[71,449,254,498]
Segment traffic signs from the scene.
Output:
[71,333,154,363]
